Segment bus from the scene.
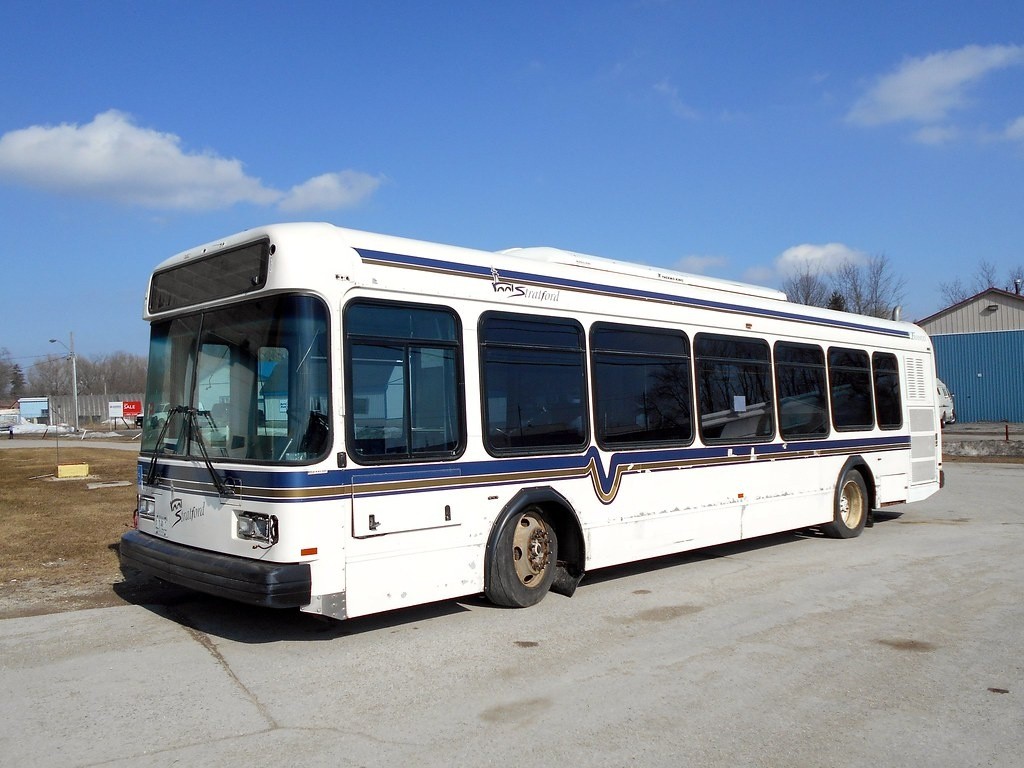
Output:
[119,221,944,621]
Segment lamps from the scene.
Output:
[988,304,998,311]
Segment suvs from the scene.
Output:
[136,403,208,428]
[211,403,264,428]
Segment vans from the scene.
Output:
[934,377,957,425]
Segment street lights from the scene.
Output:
[49,339,79,433]
